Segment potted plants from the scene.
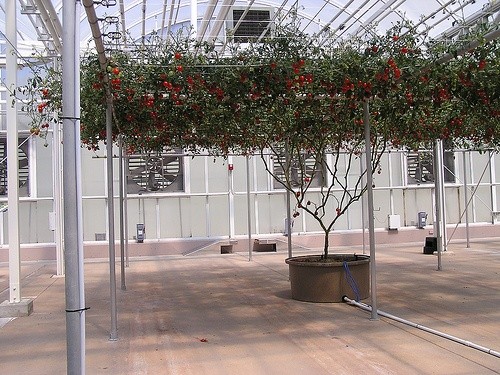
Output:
[260,129,386,304]
